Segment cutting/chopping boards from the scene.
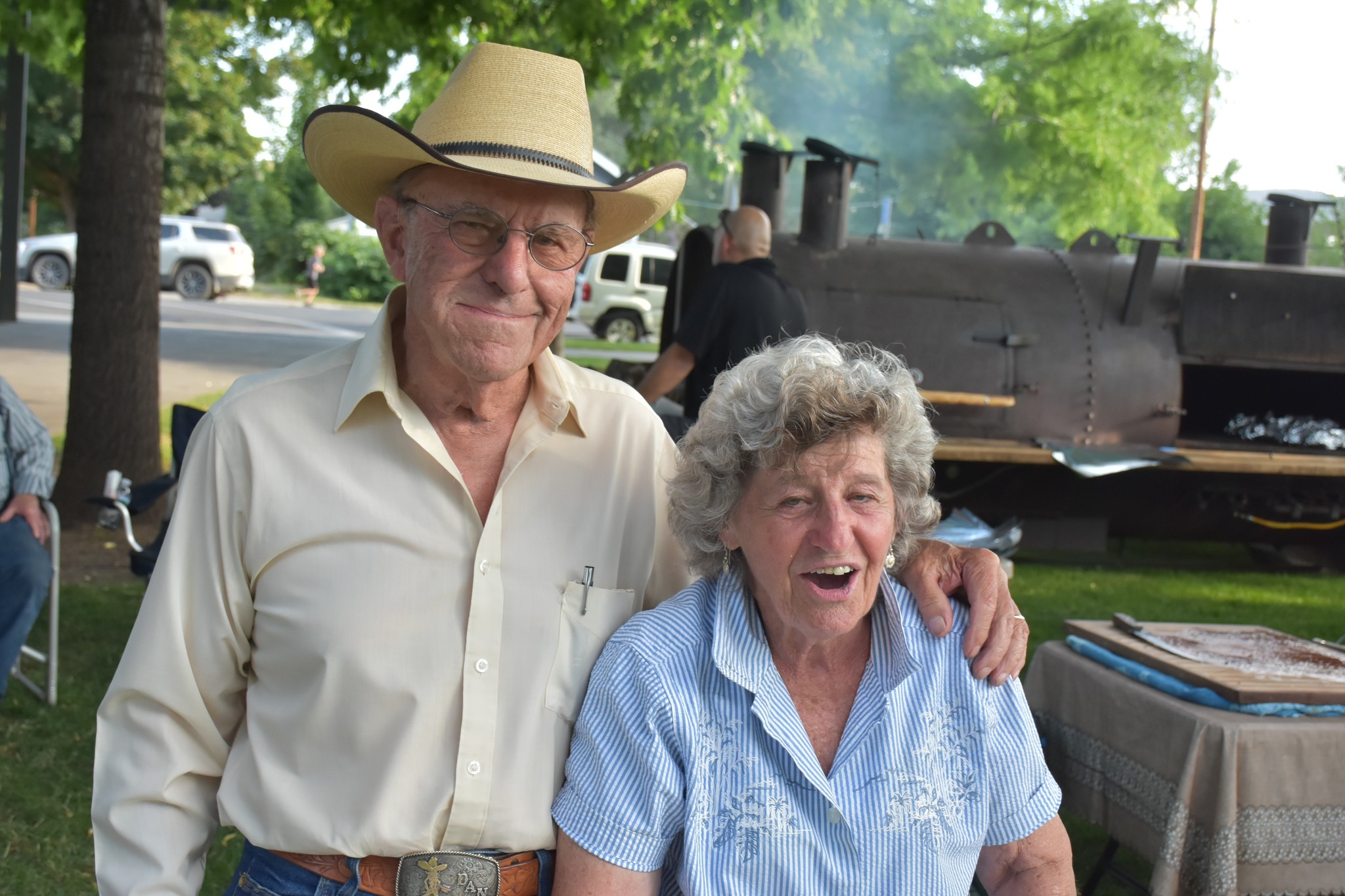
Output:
[1064,620,1345,703]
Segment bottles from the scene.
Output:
[101,478,132,530]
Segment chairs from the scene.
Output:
[6,496,61,708]
[100,400,209,593]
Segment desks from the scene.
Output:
[1020,636,1345,896]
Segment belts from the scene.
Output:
[268,849,539,896]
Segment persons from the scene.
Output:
[0,375,55,696]
[91,42,1029,896]
[635,205,807,444]
[294,245,325,311]
[549,335,1077,896]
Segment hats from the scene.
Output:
[301,41,689,258]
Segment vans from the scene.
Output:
[575,240,677,348]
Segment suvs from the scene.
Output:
[16,212,255,302]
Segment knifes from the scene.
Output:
[1113,611,1199,662]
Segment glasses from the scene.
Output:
[720,209,735,239]
[404,197,595,272]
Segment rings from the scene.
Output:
[1014,615,1025,620]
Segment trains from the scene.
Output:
[597,134,1345,576]
